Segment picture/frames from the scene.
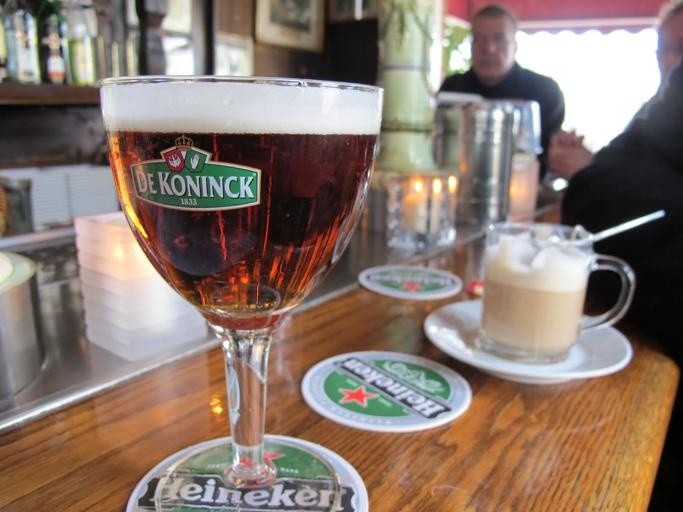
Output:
[250,0,324,56]
[213,30,256,77]
[325,0,385,27]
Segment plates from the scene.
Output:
[0,162,120,238]
[422,296,632,387]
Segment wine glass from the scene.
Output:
[97,73,384,512]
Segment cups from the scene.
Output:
[509,155,538,216]
[476,221,639,363]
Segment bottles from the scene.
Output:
[0,0,144,86]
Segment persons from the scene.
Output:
[434,4,565,200]
[548,0,683,512]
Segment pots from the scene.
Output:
[0,250,50,402]
